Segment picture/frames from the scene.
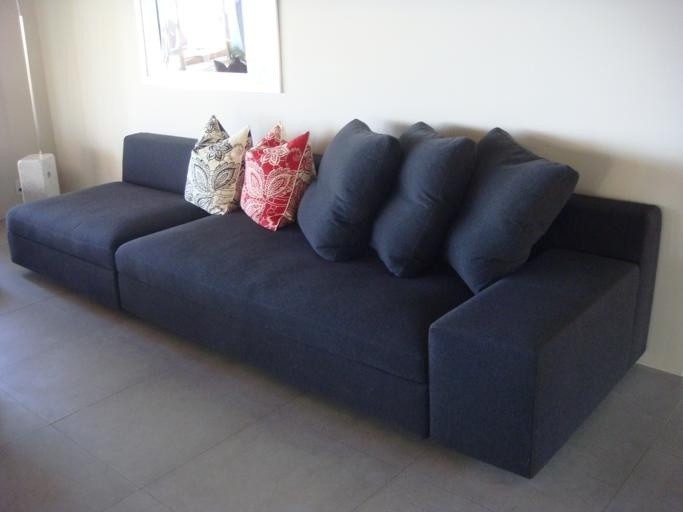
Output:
[137,0,282,94]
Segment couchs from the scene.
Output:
[5,131,663,481]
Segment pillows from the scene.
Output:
[239,123,317,232]
[364,122,477,278]
[440,126,580,296]
[297,118,403,261]
[184,115,253,216]
